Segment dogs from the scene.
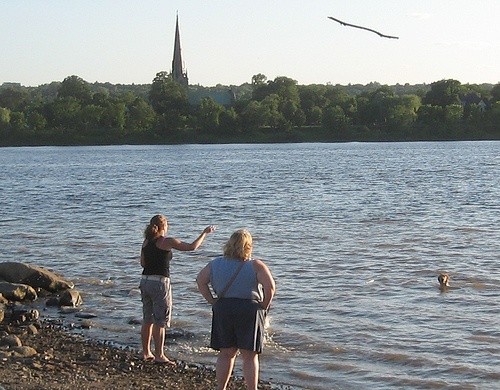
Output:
[438,274,450,287]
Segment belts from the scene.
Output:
[142,274,170,284]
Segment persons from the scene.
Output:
[194,230,276,390]
[140,215,217,364]
